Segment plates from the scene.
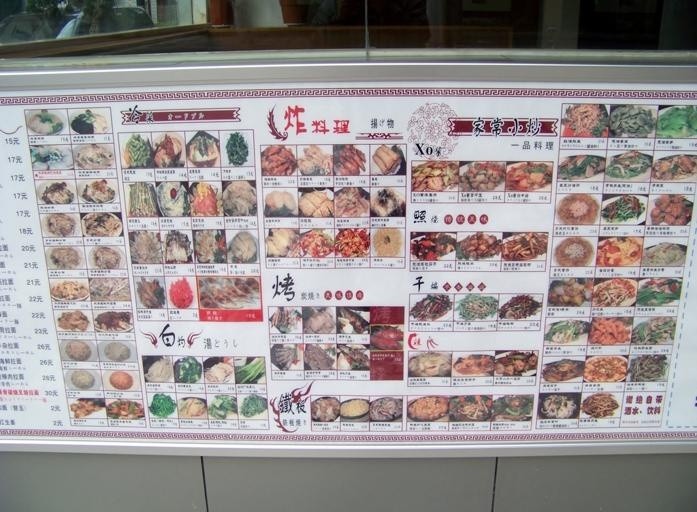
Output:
[41,212,123,236]
[263,186,406,217]
[271,343,369,370]
[553,234,689,268]
[63,369,142,393]
[35,179,120,203]
[270,305,370,333]
[408,394,534,422]
[266,226,406,257]
[147,391,268,420]
[141,354,266,383]
[25,109,112,135]
[559,104,697,138]
[68,397,146,420]
[556,149,696,183]
[127,180,258,217]
[52,308,135,333]
[410,232,548,261]
[50,279,131,300]
[30,144,116,170]
[57,340,138,362]
[128,228,261,264]
[542,354,671,382]
[539,393,623,424]
[371,323,403,350]
[550,276,681,308]
[555,193,694,226]
[260,144,406,175]
[409,292,543,321]
[44,247,127,270]
[117,132,254,167]
[134,274,262,310]
[408,350,538,378]
[411,160,553,191]
[311,395,403,423]
[546,316,677,345]
